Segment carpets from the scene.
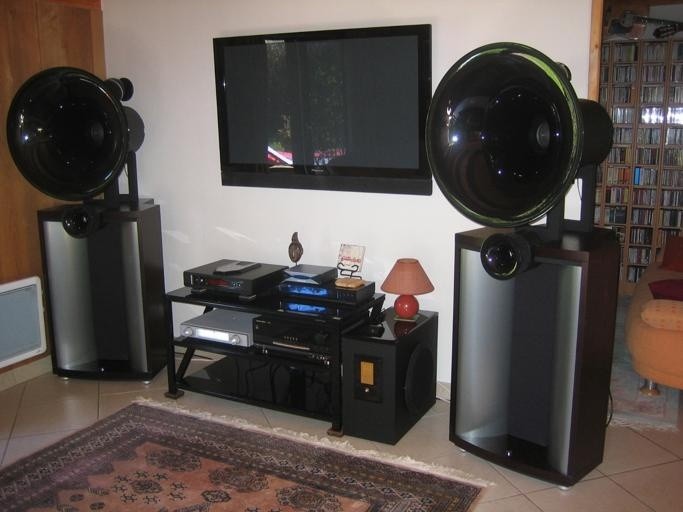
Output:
[608,309,680,432]
[0,394,499,512]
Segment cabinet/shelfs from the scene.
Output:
[593,37,683,298]
[163,287,386,437]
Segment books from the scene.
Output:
[592,45,683,283]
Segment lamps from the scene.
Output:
[381,258,435,323]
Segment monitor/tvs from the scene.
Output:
[209,23,434,197]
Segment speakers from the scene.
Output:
[4,65,165,385]
[427,42,624,489]
[341,306,439,446]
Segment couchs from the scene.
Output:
[626,242,683,397]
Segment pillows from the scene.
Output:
[658,234,683,273]
[640,299,683,332]
[648,278,683,301]
